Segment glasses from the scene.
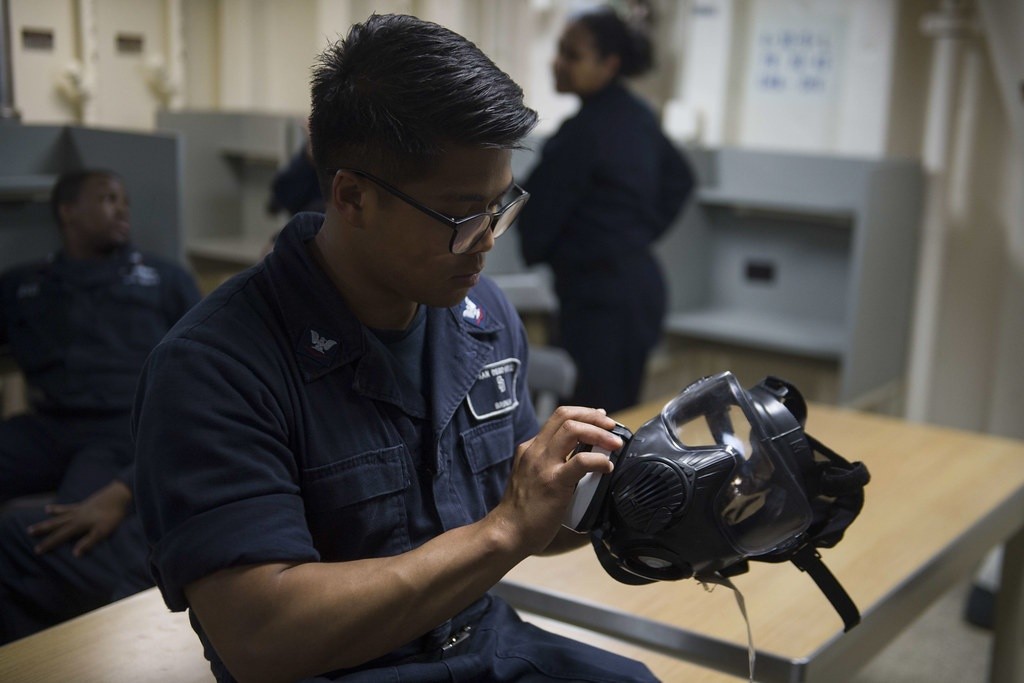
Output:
[348,168,532,256]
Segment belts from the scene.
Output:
[331,621,481,678]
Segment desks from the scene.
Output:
[1,586,754,683]
[493,390,1023,683]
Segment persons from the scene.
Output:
[512,4,697,420]
[1,169,207,649]
[132,8,665,683]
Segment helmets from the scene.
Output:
[558,368,873,587]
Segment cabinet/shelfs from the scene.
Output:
[167,108,922,414]
